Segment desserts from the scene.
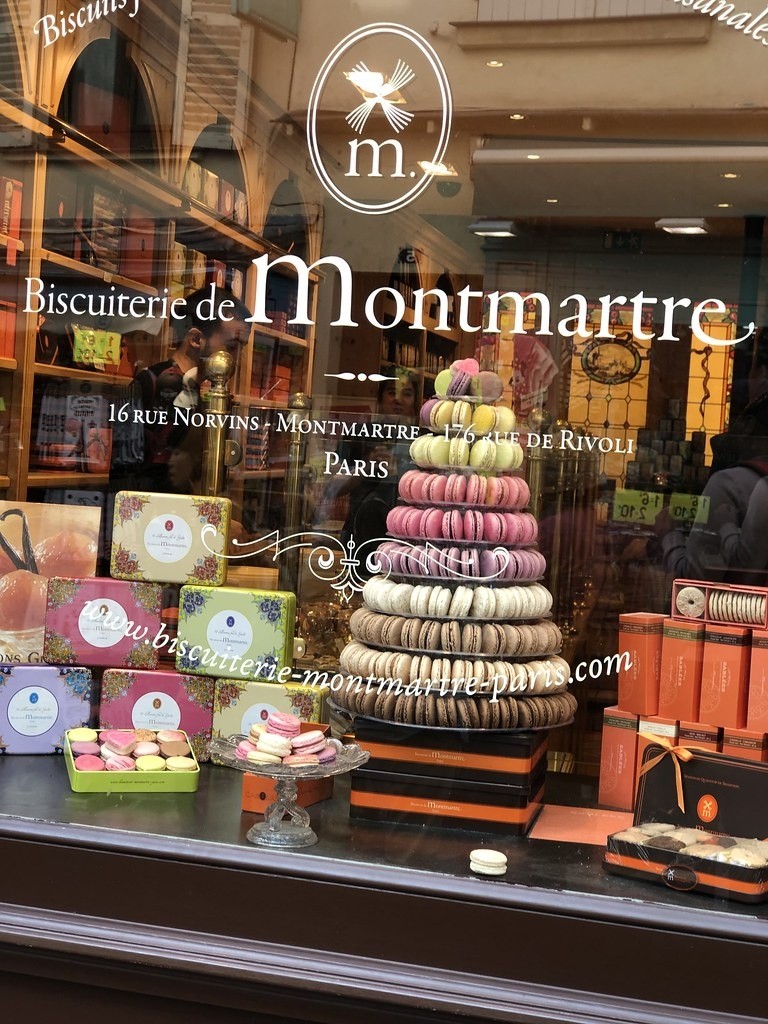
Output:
[0,508,98,661]
[68,727,198,772]
[469,849,507,875]
[329,575,578,729]
[612,821,768,868]
[676,587,767,625]
[374,358,546,580]
[234,712,337,767]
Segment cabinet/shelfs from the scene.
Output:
[334,272,463,441]
[0,86,325,590]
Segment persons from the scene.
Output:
[732,327,768,437]
[165,418,203,496]
[682,388,768,568]
[324,368,436,594]
[104,286,253,566]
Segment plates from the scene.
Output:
[206,734,372,781]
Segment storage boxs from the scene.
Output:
[0,490,332,812]
[58,87,135,158]
[600,581,768,898]
[183,161,249,227]
[265,282,313,340]
[249,346,292,403]
[168,242,244,318]
[339,718,554,835]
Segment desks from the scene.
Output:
[0,756,768,1024]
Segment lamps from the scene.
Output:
[466,218,517,237]
[655,218,711,234]
